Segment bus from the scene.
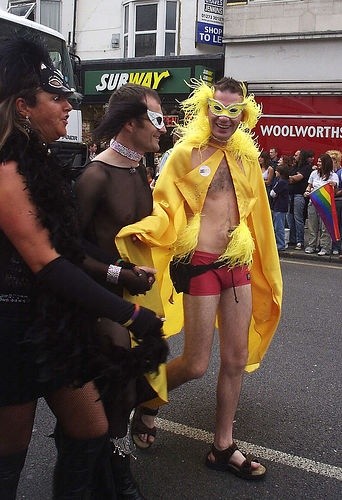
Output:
[0,10,89,178]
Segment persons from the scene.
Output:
[0,38,163,500]
[88,140,98,160]
[54,84,167,500]
[258,148,342,256]
[115,76,283,480]
[146,132,183,191]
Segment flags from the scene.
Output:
[309,183,341,241]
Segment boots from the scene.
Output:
[48,422,117,500]
[109,431,146,500]
[0,447,29,500]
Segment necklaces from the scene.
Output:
[110,138,142,162]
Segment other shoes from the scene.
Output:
[333,250,339,255]
[305,247,316,254]
[317,249,326,255]
[294,242,304,249]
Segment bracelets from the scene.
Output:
[121,304,140,327]
[106,264,122,285]
[115,258,129,266]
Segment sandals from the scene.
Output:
[207,442,266,479]
[131,407,159,450]
